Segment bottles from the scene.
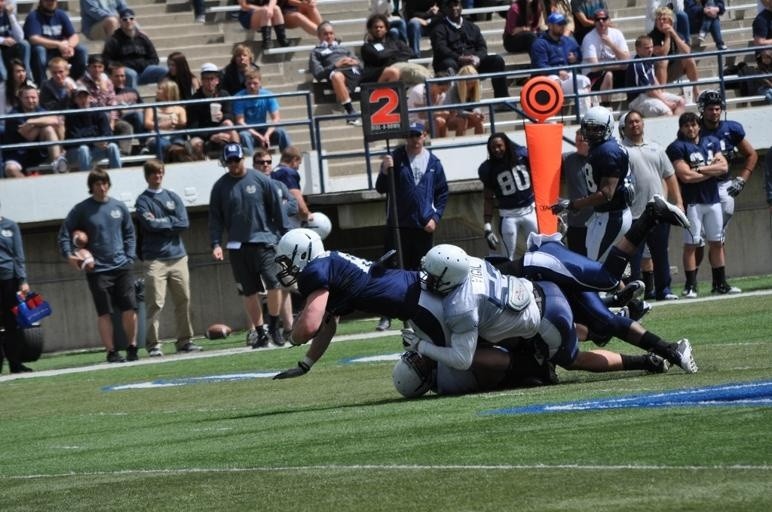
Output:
[13,289,42,319]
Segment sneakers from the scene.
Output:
[615,298,649,320]
[675,338,699,373]
[646,351,671,373]
[107,349,127,362]
[126,344,138,361]
[646,194,691,229]
[711,286,742,294]
[613,280,646,308]
[246,330,292,348]
[375,316,391,331]
[149,348,164,357]
[682,284,697,298]
[595,333,613,347]
[347,120,362,127]
[656,286,678,300]
[178,342,203,351]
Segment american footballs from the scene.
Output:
[203,323,234,340]
[70,248,96,272]
[71,229,89,250]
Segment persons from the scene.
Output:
[2,0,771,397]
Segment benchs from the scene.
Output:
[13,0,767,172]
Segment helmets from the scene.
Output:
[579,105,614,147]
[393,350,436,397]
[300,212,332,240]
[696,89,727,116]
[273,227,325,291]
[417,244,470,295]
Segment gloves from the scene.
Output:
[272,361,310,380]
[548,198,574,215]
[401,329,419,350]
[727,176,745,198]
[484,229,499,251]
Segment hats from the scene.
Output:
[200,63,221,75]
[71,81,89,95]
[408,121,425,134]
[592,9,608,19]
[223,143,243,161]
[548,12,570,25]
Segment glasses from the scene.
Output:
[254,160,272,164]
[121,15,135,22]
